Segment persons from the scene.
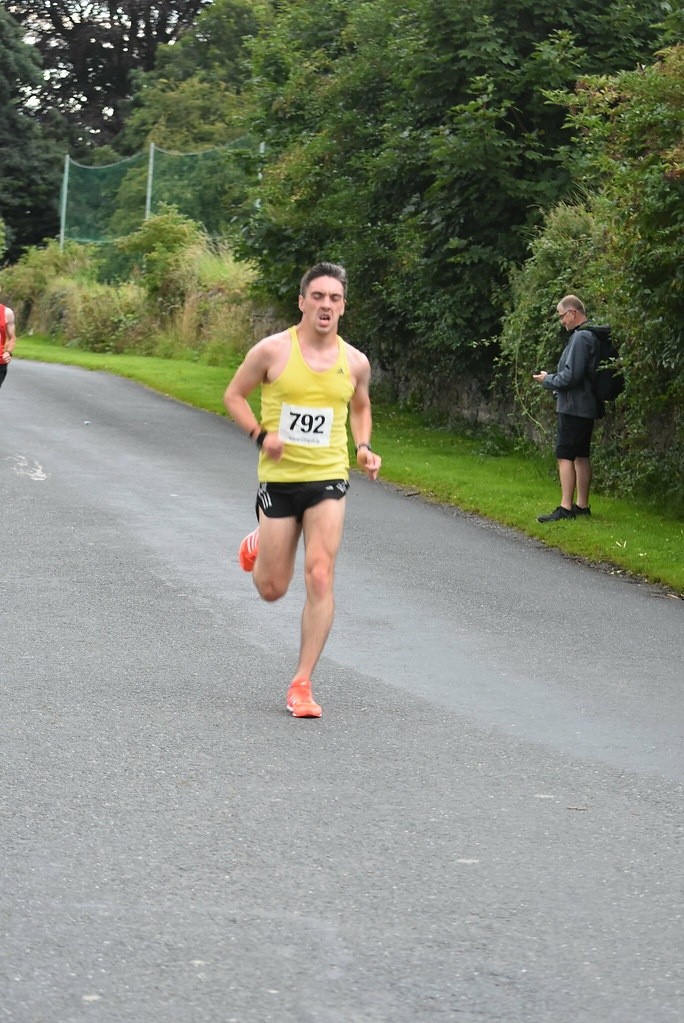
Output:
[224,262,382,718]
[533,295,607,523]
[0,280,16,388]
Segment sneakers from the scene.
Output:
[537,506,576,523]
[286,676,322,717]
[571,504,591,516]
[239,525,260,572]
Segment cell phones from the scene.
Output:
[530,371,539,375]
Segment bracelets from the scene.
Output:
[6,351,12,357]
[248,422,267,447]
[355,441,371,455]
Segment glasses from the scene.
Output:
[558,309,575,319]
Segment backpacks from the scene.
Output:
[567,328,625,401]
